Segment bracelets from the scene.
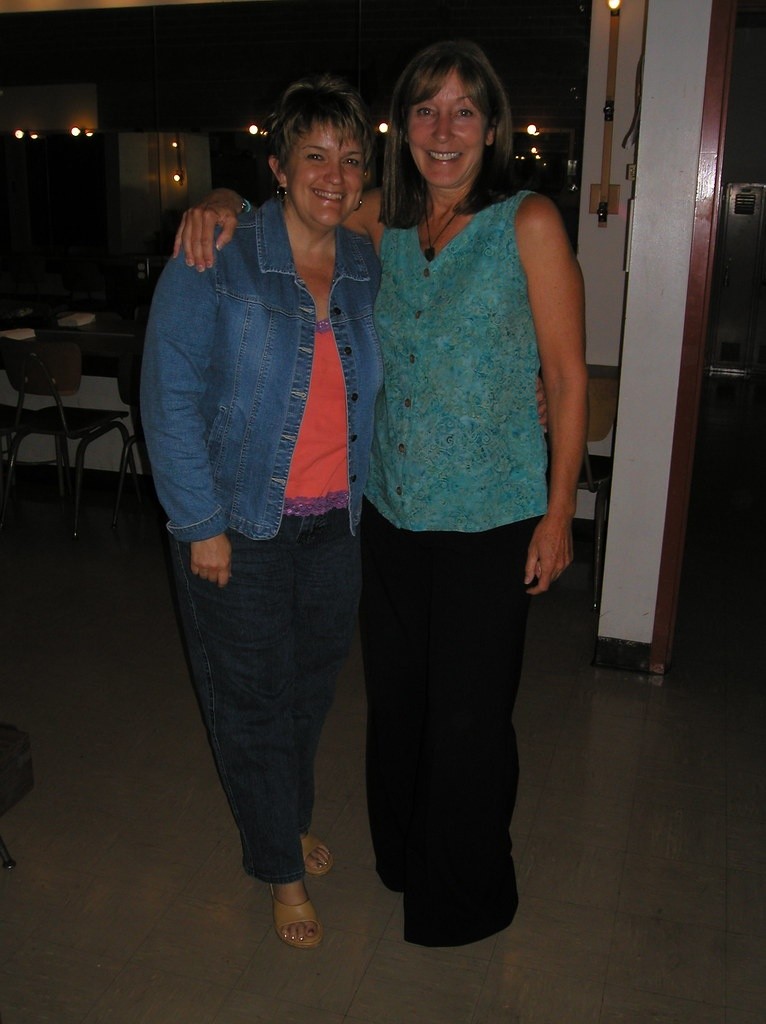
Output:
[241,198,251,213]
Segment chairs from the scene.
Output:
[0,336,140,539]
[536,363,620,611]
[110,352,155,528]
[0,337,65,497]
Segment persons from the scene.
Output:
[140,38,589,948]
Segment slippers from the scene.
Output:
[301,829,335,877]
[270,878,323,949]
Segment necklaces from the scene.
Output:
[424,191,458,261]
